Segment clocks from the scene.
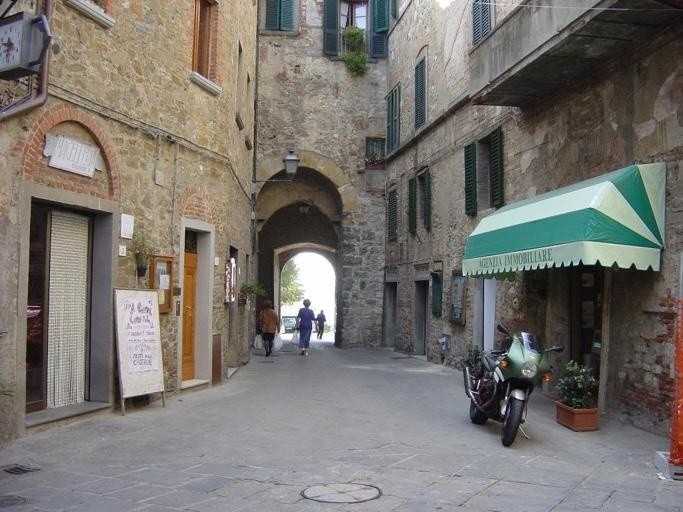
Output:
[0,11,52,81]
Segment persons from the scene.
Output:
[314,309,326,338]
[295,298,317,357]
[257,298,280,357]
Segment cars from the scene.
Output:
[282,318,300,332]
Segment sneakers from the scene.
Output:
[299,347,309,357]
[266,350,272,357]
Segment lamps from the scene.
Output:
[255,150,300,183]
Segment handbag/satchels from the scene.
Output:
[270,333,284,352]
[253,333,263,351]
[291,328,298,346]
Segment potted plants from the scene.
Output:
[554,360,598,433]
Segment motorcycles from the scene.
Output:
[463,322,565,447]
